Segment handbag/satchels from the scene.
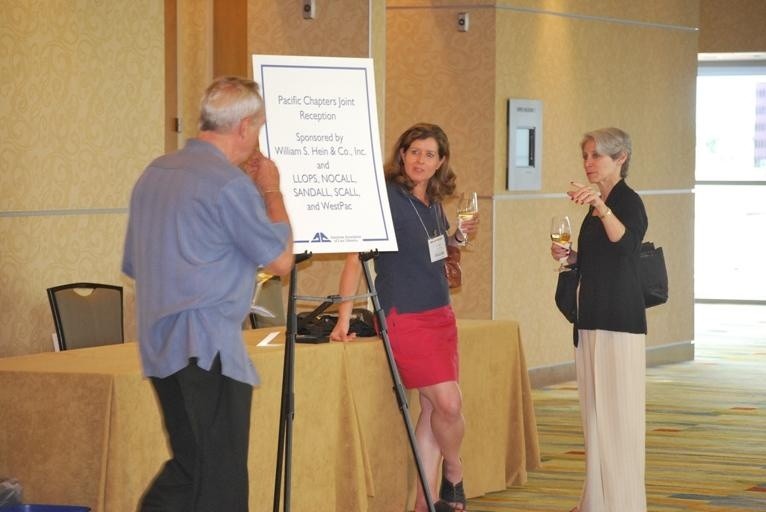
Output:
[554,242,668,323]
[442,246,462,288]
[296,296,376,344]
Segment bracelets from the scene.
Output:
[601,207,612,220]
[454,233,464,243]
[262,189,280,196]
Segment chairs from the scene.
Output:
[45,281,125,351]
[248,273,285,329]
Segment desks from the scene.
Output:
[0,317,546,512]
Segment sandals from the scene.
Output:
[439,458,465,512]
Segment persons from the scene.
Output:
[330,122,481,512]
[120,75,296,512]
[551,127,648,512]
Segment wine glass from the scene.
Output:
[455,189,480,246]
[548,214,573,274]
